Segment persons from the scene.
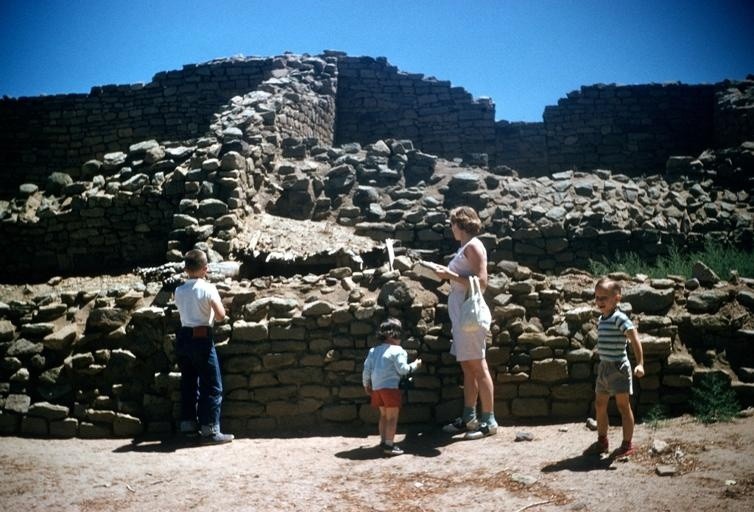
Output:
[432,203,501,441]
[582,276,646,461]
[173,247,237,446]
[362,316,423,456]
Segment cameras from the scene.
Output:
[399,378,416,390]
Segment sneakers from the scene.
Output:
[378,442,404,456]
[181,430,235,446]
[582,441,609,457]
[610,446,634,458]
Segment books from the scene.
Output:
[417,258,441,271]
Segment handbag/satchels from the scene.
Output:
[458,274,491,334]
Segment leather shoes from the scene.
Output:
[463,423,498,441]
[442,418,479,433]
